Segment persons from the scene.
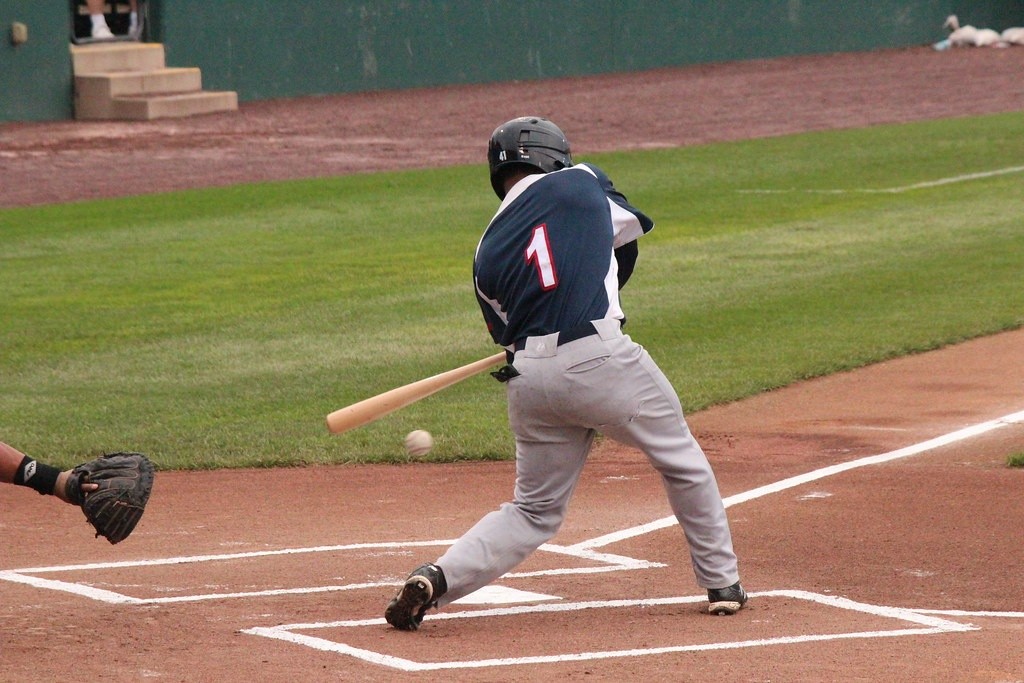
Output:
[385,117,748,633]
[0,441,98,507]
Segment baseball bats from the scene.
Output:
[324,352,509,435]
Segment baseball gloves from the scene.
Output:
[65,450,155,545]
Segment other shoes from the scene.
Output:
[90,22,116,38]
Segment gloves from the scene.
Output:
[491,365,518,382]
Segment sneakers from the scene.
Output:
[386,562,447,630]
[708,582,747,614]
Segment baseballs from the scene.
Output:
[406,430,432,457]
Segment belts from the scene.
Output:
[514,321,597,353]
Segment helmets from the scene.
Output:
[487,117,572,202]
